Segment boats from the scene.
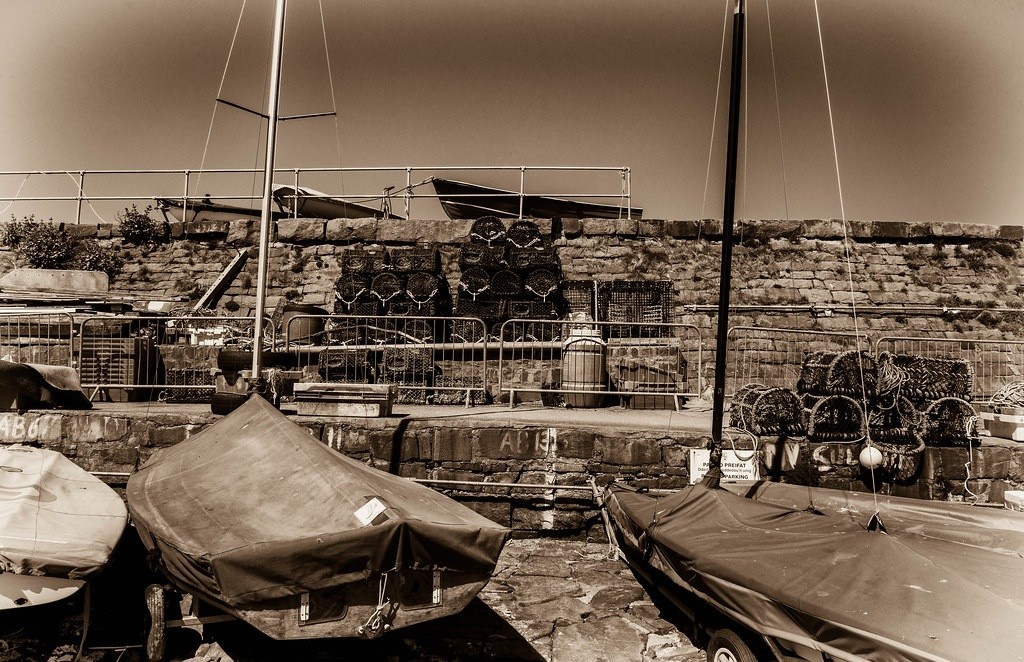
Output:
[155,195,298,221]
[0,439,127,616]
[271,182,407,221]
[430,176,647,224]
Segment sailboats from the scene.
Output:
[120,0,509,642]
[590,0,1024,662]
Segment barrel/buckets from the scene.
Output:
[281,311,323,346]
[562,329,607,407]
